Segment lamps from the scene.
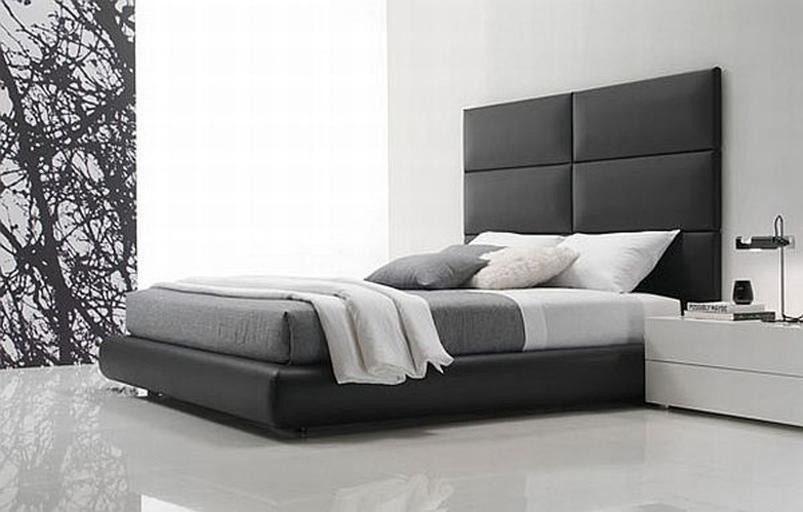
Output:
[736,217,802,324]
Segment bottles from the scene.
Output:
[732,280,754,305]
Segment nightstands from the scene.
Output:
[644,316,803,429]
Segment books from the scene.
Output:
[687,300,765,313]
[682,309,776,323]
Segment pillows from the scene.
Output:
[555,230,682,291]
[466,243,581,291]
[469,231,568,249]
[442,244,507,261]
[364,253,490,290]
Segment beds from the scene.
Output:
[96,67,721,439]
